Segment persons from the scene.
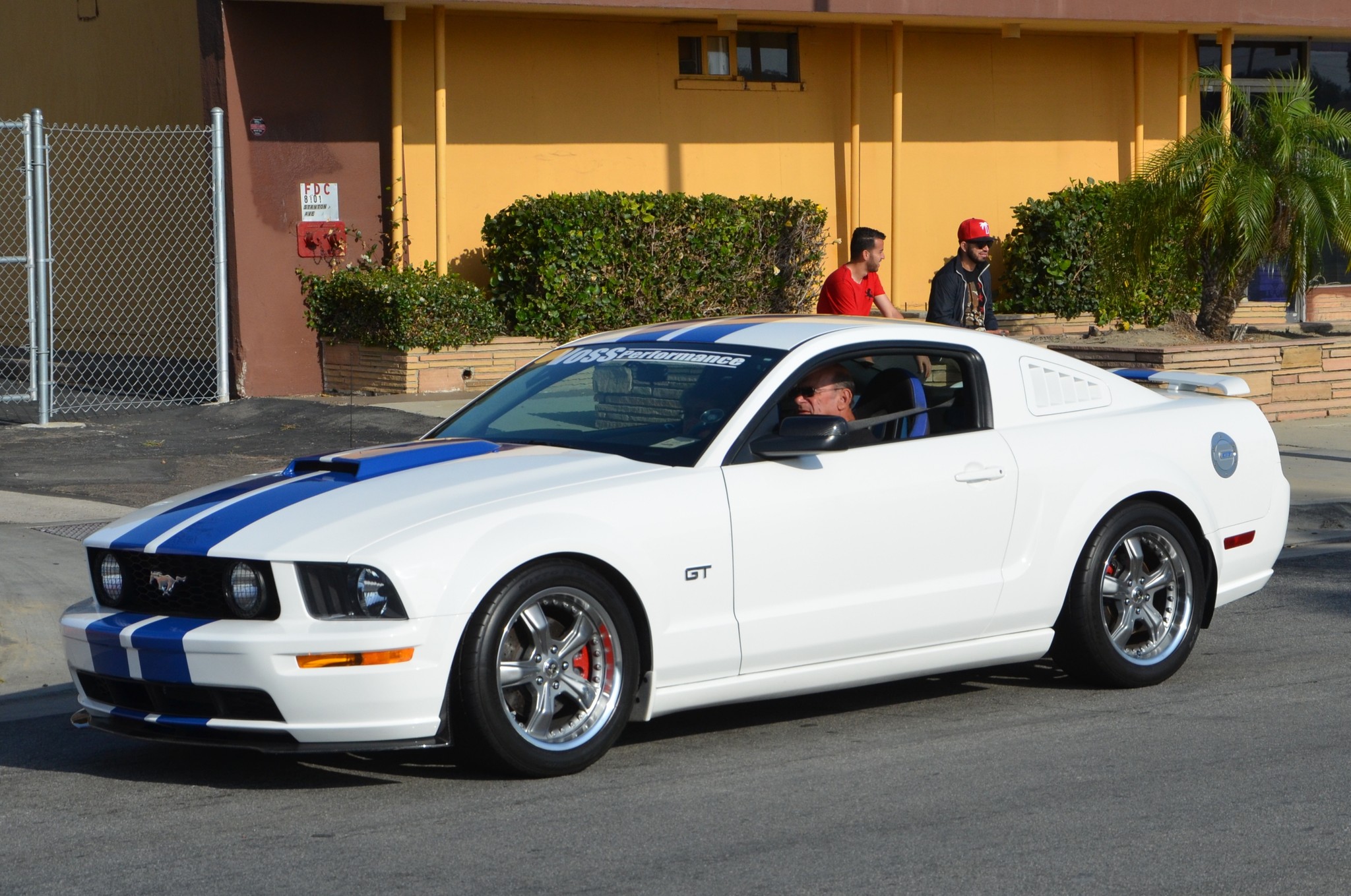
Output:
[817,227,932,385]
[795,364,876,444]
[925,218,1010,372]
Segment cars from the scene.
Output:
[58,313,1290,778]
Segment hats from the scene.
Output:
[958,218,997,241]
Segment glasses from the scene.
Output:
[966,238,994,249]
[786,386,844,398]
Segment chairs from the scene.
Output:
[838,360,931,442]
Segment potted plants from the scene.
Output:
[1306,272,1351,323]
[295,176,561,399]
